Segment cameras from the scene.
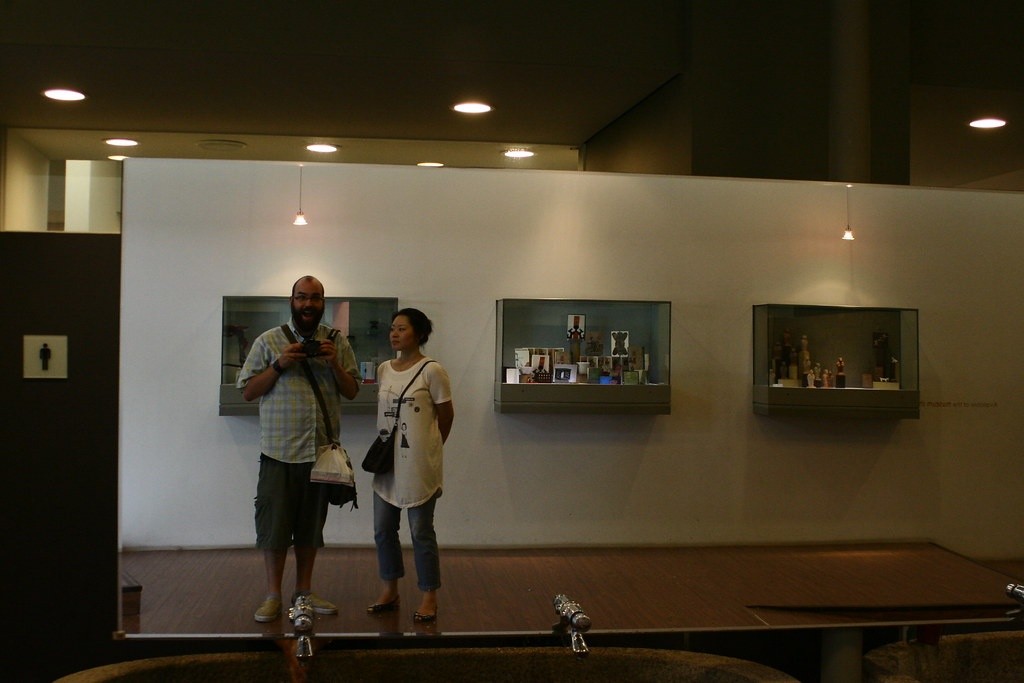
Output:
[299,339,326,358]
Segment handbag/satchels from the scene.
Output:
[361,423,399,473]
[308,441,359,511]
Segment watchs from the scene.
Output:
[272,360,284,373]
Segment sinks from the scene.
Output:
[49,643,799,683]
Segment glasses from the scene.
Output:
[292,295,325,304]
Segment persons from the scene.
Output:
[235,276,363,622]
[774,327,845,388]
[369,308,455,620]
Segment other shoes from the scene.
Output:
[294,592,339,615]
[253,598,282,622]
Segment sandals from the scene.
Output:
[366,594,401,613]
[414,602,439,623]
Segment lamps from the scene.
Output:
[841,189,855,241]
[292,167,309,226]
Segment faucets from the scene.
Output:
[289,595,315,612]
[551,590,574,631]
[562,604,598,662]
[284,608,315,657]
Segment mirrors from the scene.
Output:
[118,158,1024,639]
